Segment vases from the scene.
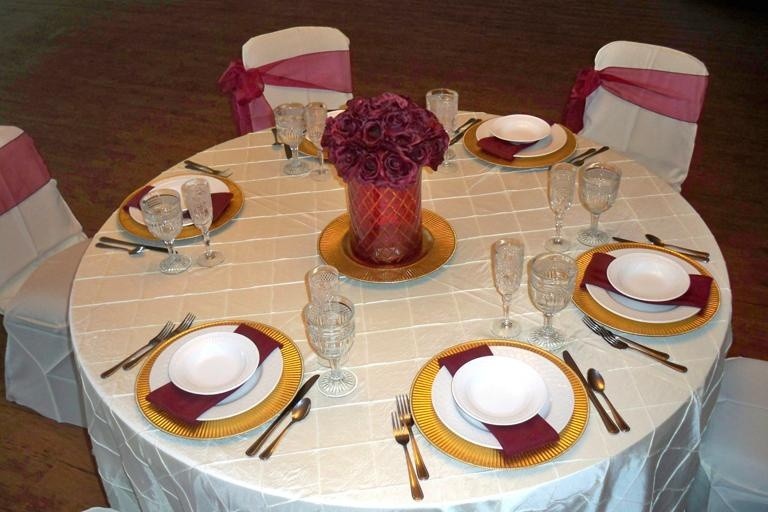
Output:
[349,176,421,266]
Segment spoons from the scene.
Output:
[271,128,282,146]
[257,397,312,460]
[587,367,631,433]
[95,242,144,256]
[561,146,610,167]
[644,233,711,259]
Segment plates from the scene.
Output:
[409,338,588,468]
[465,114,577,172]
[567,241,720,336]
[118,174,246,240]
[131,319,303,440]
[294,107,348,161]
[314,204,457,285]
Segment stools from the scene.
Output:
[699,355,768,511]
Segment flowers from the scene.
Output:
[319,92,448,186]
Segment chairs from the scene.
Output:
[218,25,354,136]
[0,125,96,427]
[574,40,709,193]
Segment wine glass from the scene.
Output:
[490,237,526,338]
[303,265,358,397]
[544,162,578,252]
[528,251,579,351]
[575,162,621,247]
[425,88,460,175]
[181,179,226,267]
[274,101,333,182]
[140,189,191,274]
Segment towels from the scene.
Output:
[145,321,283,426]
[123,186,233,225]
[476,136,539,161]
[433,343,562,460]
[579,250,713,322]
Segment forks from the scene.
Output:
[183,160,233,178]
[581,315,688,373]
[389,412,425,500]
[594,326,690,375]
[394,393,430,480]
[100,321,173,382]
[123,311,196,371]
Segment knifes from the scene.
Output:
[99,236,178,254]
[561,350,619,433]
[284,143,292,159]
[245,373,321,456]
[611,237,709,263]
[447,117,482,145]
